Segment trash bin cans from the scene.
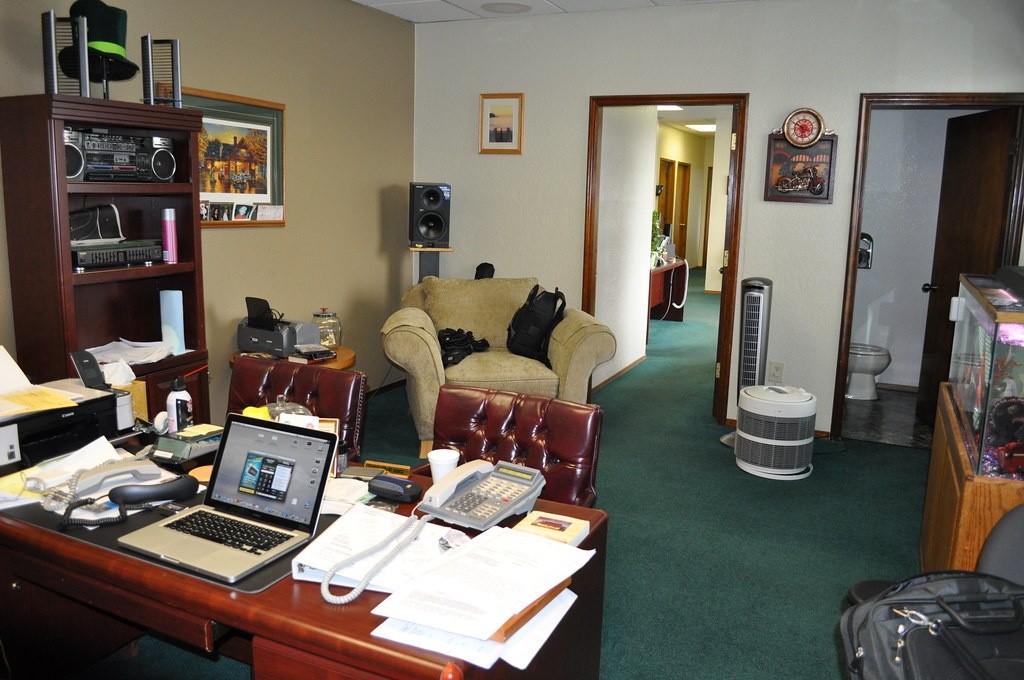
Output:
[734,384,818,480]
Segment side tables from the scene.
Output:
[228,345,357,370]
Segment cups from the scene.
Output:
[428,449,460,484]
[161,208,178,264]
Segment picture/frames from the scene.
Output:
[763,133,838,204]
[155,81,288,229]
[478,93,523,155]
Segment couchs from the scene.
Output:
[381,275,618,459]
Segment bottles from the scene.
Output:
[312,308,342,349]
[166,375,193,434]
[263,395,312,421]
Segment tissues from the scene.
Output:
[98,358,149,424]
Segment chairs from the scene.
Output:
[432,383,605,509]
[226,354,368,463]
[845,504,1024,606]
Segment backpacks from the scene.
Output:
[507,284,566,370]
[840,570,1024,680]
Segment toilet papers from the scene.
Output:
[159,290,186,352]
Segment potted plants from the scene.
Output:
[650,210,666,270]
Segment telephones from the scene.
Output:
[417,458,547,533]
[108,474,199,506]
[70,460,162,497]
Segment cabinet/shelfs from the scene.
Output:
[0,93,211,445]
[646,261,687,344]
[917,380,1024,573]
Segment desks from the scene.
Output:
[0,419,609,680]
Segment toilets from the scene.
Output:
[846,342,891,400]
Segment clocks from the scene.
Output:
[783,108,826,150]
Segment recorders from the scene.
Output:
[63,127,176,183]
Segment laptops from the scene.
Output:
[118,413,339,584]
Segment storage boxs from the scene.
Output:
[947,271,1024,481]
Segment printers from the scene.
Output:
[0,344,118,478]
[237,296,320,358]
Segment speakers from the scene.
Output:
[409,182,451,248]
